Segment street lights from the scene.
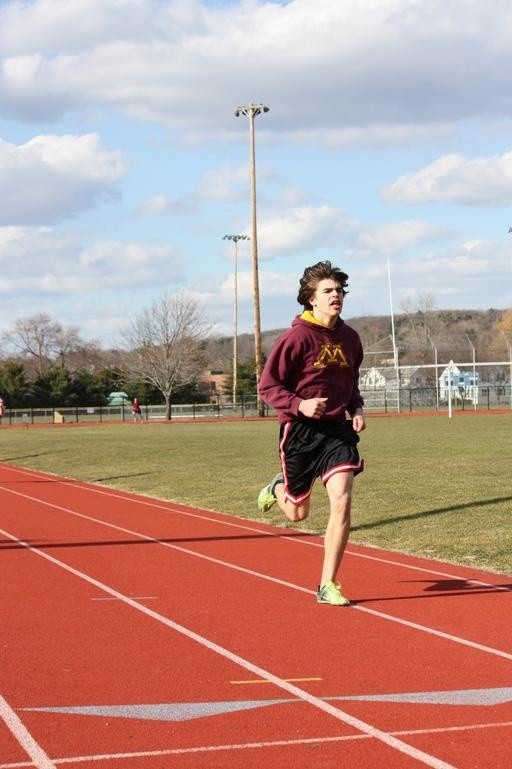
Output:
[222,232,251,408]
[233,100,270,418]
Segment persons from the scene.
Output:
[132,397,143,424]
[253,259,366,606]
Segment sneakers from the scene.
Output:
[317,581,350,606]
[257,472,285,513]
